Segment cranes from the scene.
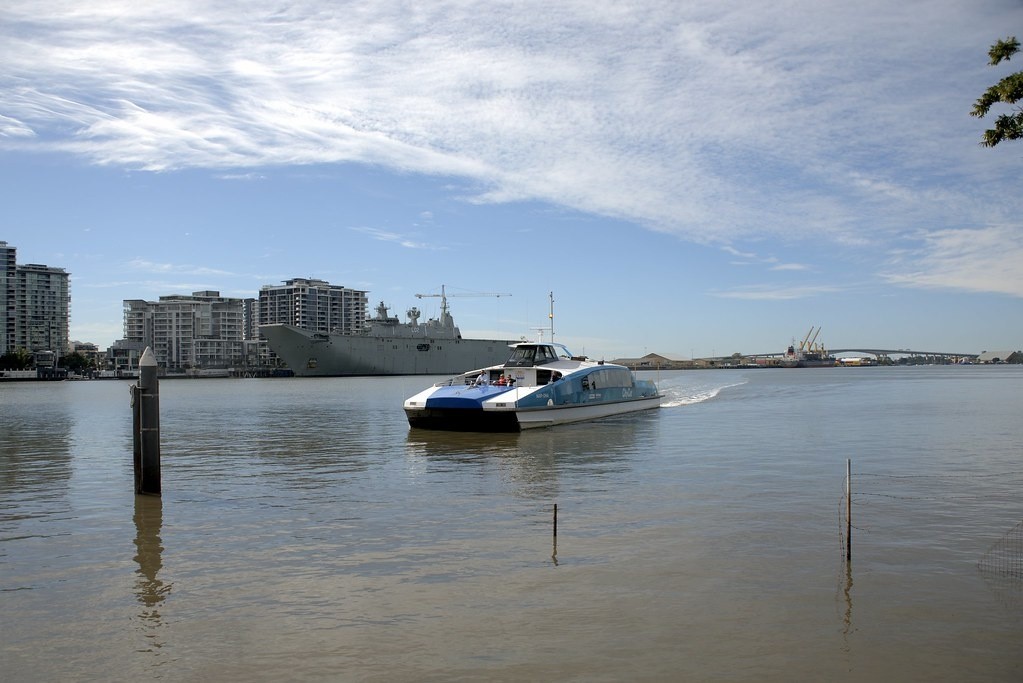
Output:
[414,284,513,313]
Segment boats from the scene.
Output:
[778,325,838,369]
[403,340,667,435]
[840,356,879,367]
[255,295,540,378]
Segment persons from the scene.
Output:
[475,370,489,386]
[493,374,506,386]
[504,375,516,386]
